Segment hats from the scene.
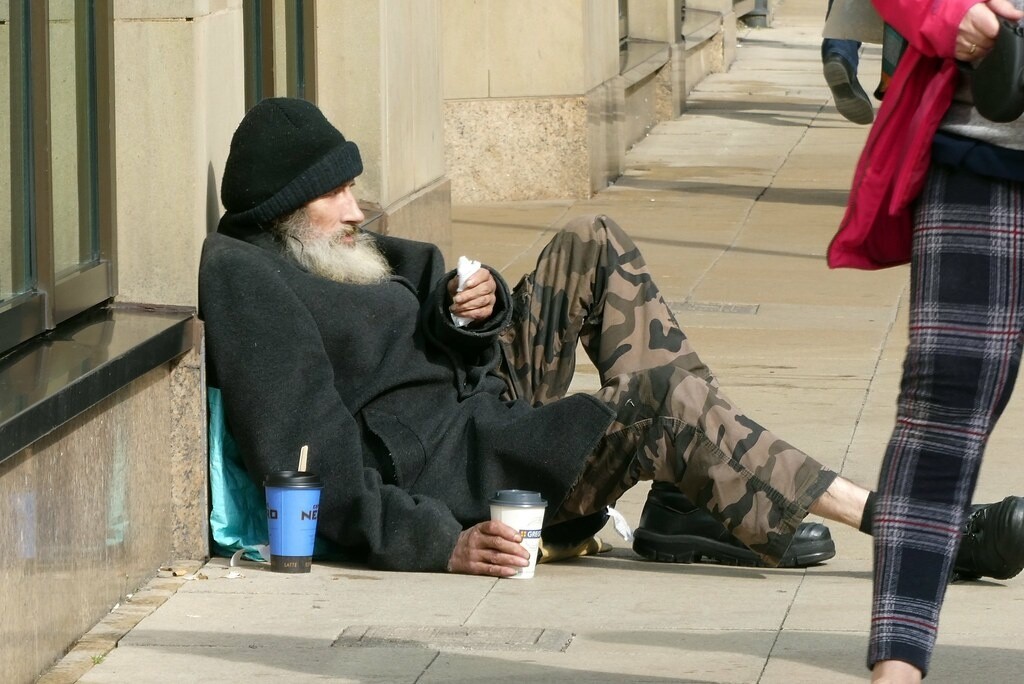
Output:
[220,97,364,237]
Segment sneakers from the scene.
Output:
[953,496,1024,581]
[632,483,835,567]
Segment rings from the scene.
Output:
[967,42,978,55]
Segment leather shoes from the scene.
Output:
[824,53,874,125]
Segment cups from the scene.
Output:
[488,489,548,579]
[262,470,325,573]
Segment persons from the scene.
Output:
[824,1,1022,681]
[820,3,909,123]
[200,97,1022,577]
